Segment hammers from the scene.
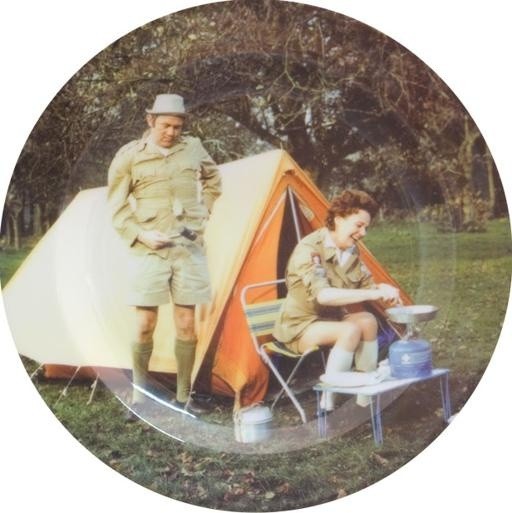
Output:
[168,226,198,241]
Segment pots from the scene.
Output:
[233,404,274,443]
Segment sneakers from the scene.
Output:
[172,398,211,414]
[124,403,143,421]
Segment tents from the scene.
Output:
[1,147,421,414]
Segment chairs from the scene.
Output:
[240,276,335,426]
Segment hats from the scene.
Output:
[144,93,193,119]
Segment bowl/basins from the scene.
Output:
[384,305,441,324]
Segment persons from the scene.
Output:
[107,94,224,422]
[271,188,404,420]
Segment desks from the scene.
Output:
[311,366,451,450]
